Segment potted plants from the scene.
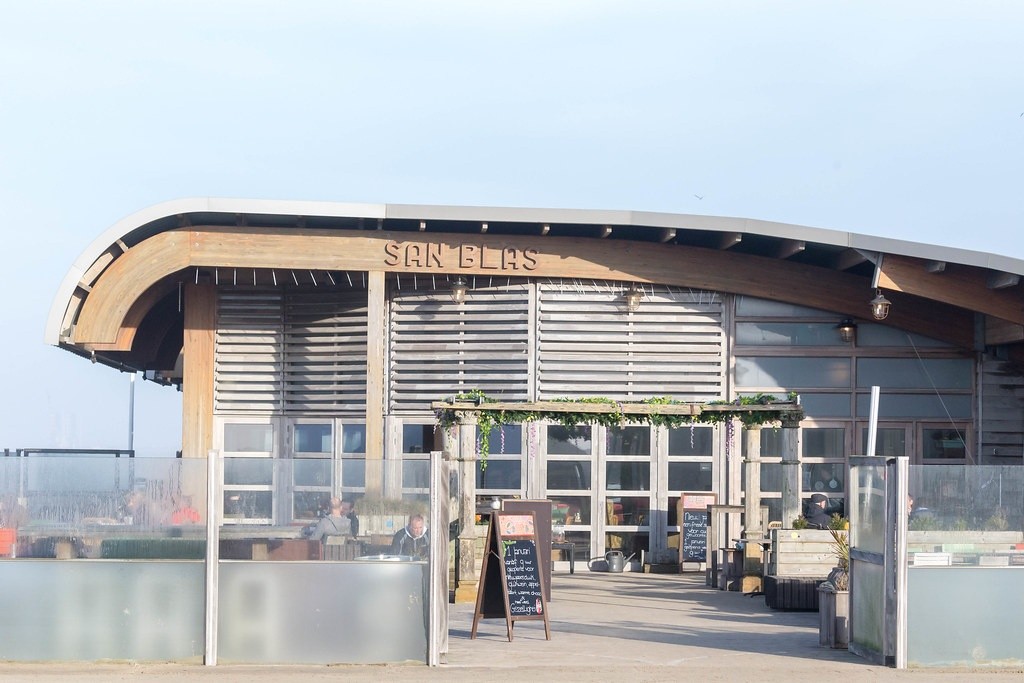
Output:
[826,526,850,591]
[772,504,1024,575]
[354,494,427,536]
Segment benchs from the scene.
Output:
[764,575,827,612]
[101,540,271,560]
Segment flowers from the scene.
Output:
[433,389,797,472]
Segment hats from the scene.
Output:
[811,494,827,503]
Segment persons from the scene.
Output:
[302,497,360,540]
[908,495,936,520]
[803,494,834,529]
[388,513,429,558]
[170,499,200,526]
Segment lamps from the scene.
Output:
[623,281,645,308]
[837,319,858,342]
[870,289,892,320]
[450,275,469,304]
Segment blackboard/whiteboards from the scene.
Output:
[497,537,549,620]
[682,507,707,562]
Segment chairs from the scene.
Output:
[321,533,360,561]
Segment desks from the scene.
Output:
[552,543,575,574]
[565,536,590,561]
[719,548,736,590]
[732,539,772,575]
